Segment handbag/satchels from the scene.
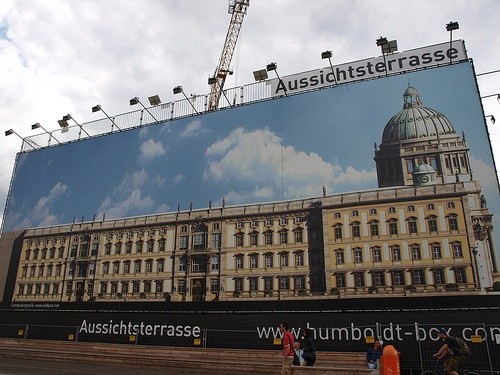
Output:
[293,350,300,366]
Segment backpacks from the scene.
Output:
[447,336,469,353]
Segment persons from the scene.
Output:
[365,340,402,369]
[292,341,307,366]
[278,322,295,375]
[302,329,317,366]
[432,327,466,375]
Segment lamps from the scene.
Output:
[376,36,388,75]
[5,129,36,150]
[92,105,121,132]
[208,78,232,107]
[481,94,500,104]
[446,21,459,65]
[32,123,60,143]
[485,115,495,124]
[130,97,159,124]
[322,51,337,85]
[63,114,91,138]
[267,62,287,97]
[173,86,199,115]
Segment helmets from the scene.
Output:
[437,328,446,335]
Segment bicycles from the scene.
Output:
[421,356,478,375]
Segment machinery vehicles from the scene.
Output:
[207,0,251,109]
